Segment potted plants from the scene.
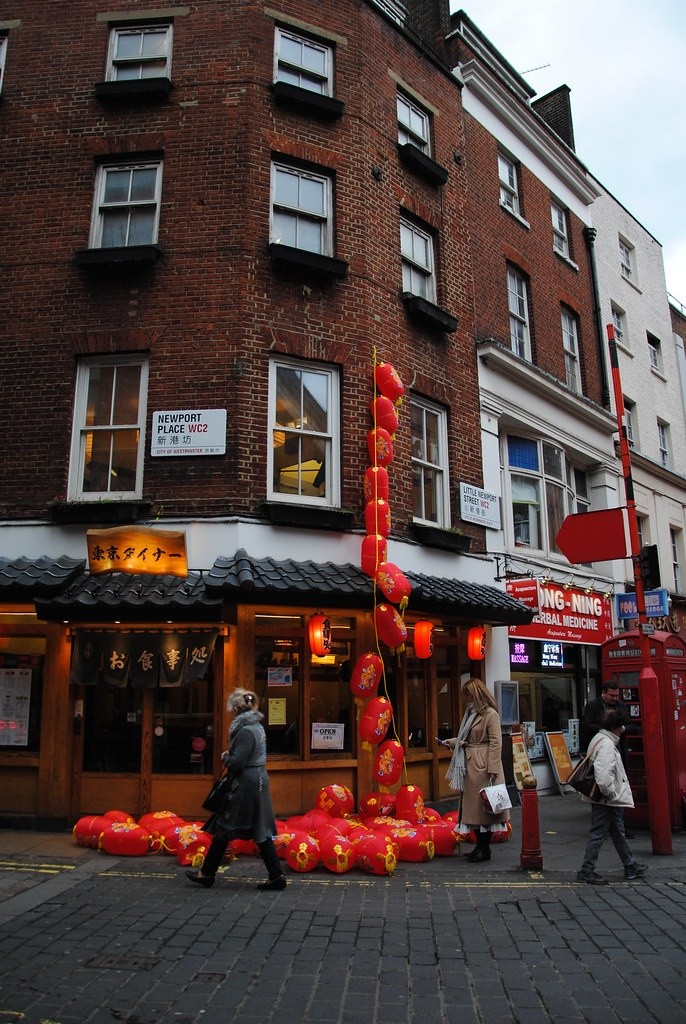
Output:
[406,296,457,333]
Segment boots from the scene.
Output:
[464,828,494,861]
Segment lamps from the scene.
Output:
[576,578,596,596]
[658,586,673,608]
[556,573,575,591]
[533,568,551,587]
[595,583,614,600]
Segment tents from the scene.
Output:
[370,395,399,441]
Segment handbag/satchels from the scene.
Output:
[567,737,609,802]
[479,778,513,814]
[202,775,232,813]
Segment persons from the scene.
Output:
[580,681,632,836]
[445,678,511,862]
[575,710,647,886]
[186,687,287,892]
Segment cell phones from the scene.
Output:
[434,736,448,746]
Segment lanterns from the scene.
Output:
[375,603,408,656]
[365,497,390,538]
[468,625,487,660]
[308,611,332,657]
[376,361,405,407]
[349,650,382,708]
[274,785,512,878]
[372,738,403,795]
[414,618,435,659]
[367,427,394,467]
[364,465,388,503]
[360,534,387,580]
[357,695,393,752]
[73,811,262,866]
[376,562,412,610]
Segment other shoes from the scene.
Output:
[186,871,217,887]
[625,862,648,880]
[576,871,608,884]
[258,874,287,889]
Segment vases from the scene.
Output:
[270,243,349,280]
[398,143,449,186]
[72,243,166,267]
[272,80,345,120]
[95,77,173,101]
